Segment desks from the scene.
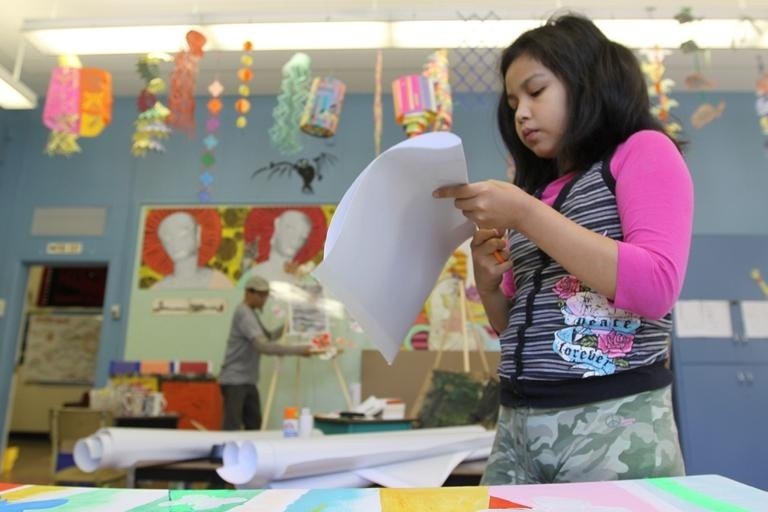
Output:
[1,475,767,511]
[127,461,222,489]
[314,414,413,436]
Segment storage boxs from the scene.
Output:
[360,347,500,417]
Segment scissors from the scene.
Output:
[471,220,511,265]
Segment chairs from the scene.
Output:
[48,407,127,488]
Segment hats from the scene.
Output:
[245,276,275,292]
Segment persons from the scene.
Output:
[148,210,223,287]
[216,277,312,431]
[434,11,694,486]
[237,207,319,290]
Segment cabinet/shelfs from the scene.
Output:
[158,380,223,430]
